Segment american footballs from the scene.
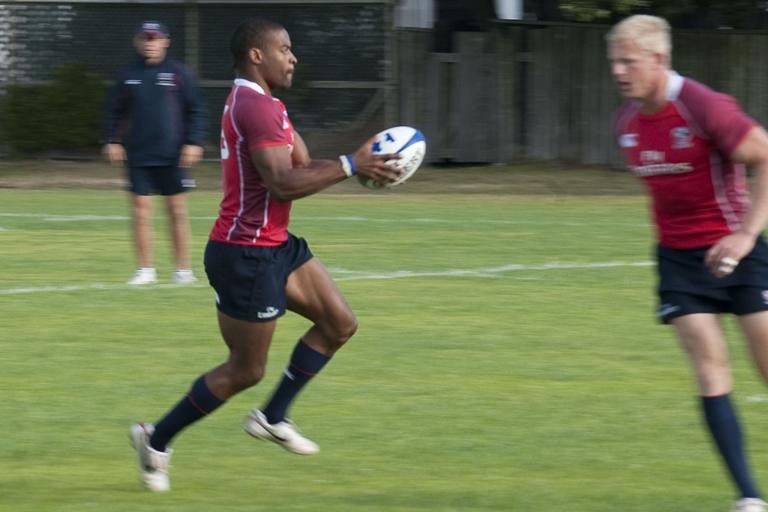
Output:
[358,126,426,190]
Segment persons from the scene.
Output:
[99,21,210,287]
[604,15,768,512]
[128,18,403,494]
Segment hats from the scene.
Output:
[134,20,171,36]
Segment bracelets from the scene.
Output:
[339,153,356,179]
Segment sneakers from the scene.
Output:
[735,495,766,512]
[241,408,320,455]
[129,421,173,491]
[174,270,197,284]
[127,268,156,284]
